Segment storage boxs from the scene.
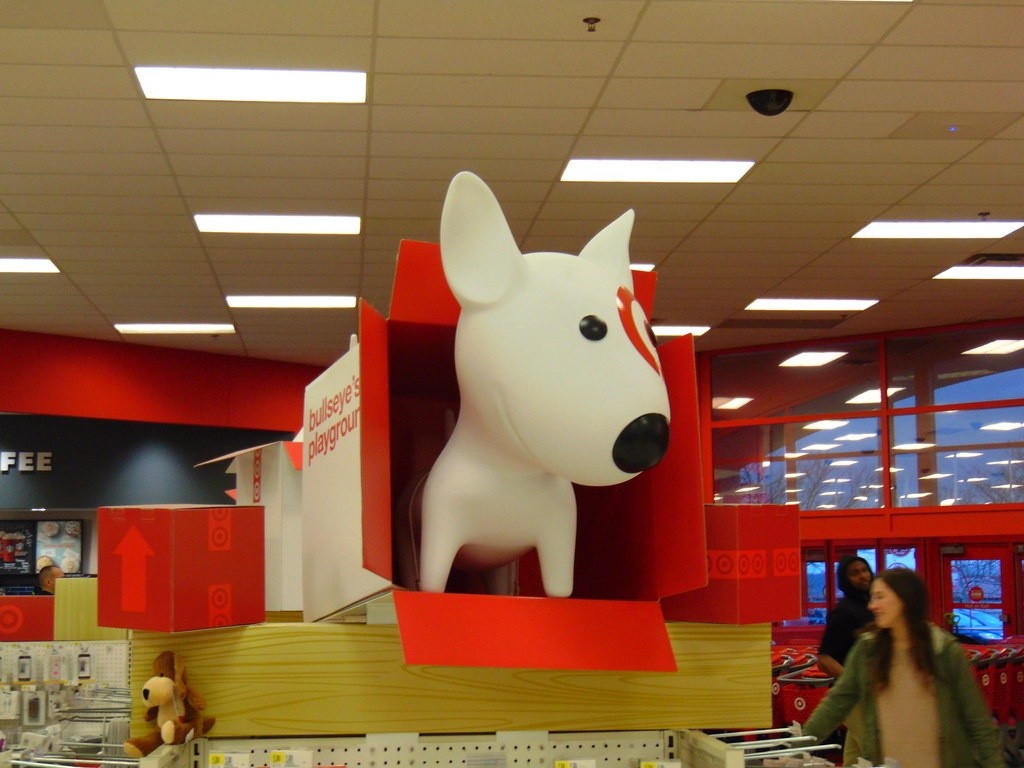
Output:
[97,502,266,633]
[54,578,127,641]
[194,440,303,612]
[302,239,707,671]
[660,502,802,624]
[0,595,53,641]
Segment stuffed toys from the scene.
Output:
[124,650,216,758]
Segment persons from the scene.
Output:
[817,556,873,680]
[801,568,1007,768]
[36,565,65,596]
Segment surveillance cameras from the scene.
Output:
[746,89,793,116]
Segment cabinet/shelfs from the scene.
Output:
[0,623,841,768]
